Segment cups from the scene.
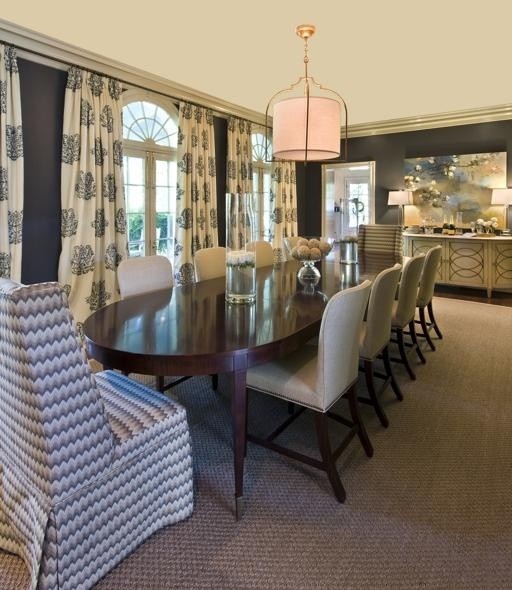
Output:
[494,229,500,236]
[424,215,432,226]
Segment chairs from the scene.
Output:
[415,242,445,354]
[191,244,234,283]
[238,277,380,504]
[243,239,273,271]
[2,273,203,589]
[280,237,308,260]
[116,252,220,402]
[354,221,406,257]
[359,258,402,432]
[391,250,427,385]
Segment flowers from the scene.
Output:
[474,216,498,232]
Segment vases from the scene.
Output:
[337,198,358,264]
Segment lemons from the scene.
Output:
[292,238,329,260]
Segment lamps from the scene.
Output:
[488,187,512,235]
[260,23,350,171]
[388,187,413,223]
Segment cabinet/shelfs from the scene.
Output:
[404,230,510,300]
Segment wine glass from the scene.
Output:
[286,283,328,316]
[470,220,476,233]
[281,236,335,280]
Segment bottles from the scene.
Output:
[448,215,455,235]
[456,210,463,235]
[442,213,449,234]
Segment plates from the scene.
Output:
[404,227,424,234]
